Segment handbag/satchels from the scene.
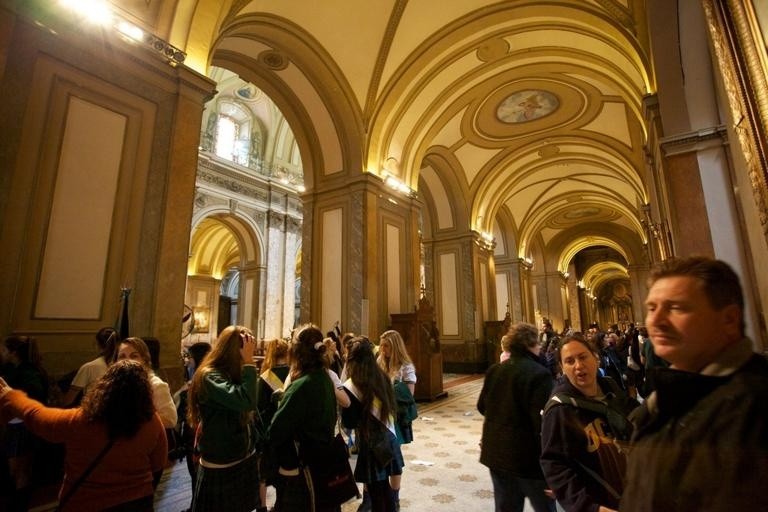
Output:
[298,432,361,512]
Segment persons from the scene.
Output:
[477,257,767,511]
[1,322,416,512]
